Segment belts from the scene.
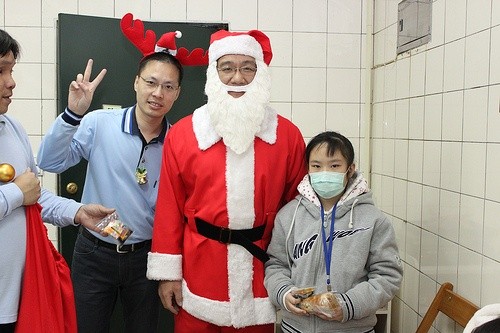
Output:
[78,226,152,253]
[184,214,270,263]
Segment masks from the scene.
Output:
[308,166,350,199]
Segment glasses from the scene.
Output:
[218,65,257,73]
[139,75,180,92]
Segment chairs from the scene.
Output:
[415,282,500,333]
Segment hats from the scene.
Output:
[208,29,273,62]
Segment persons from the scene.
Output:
[146,28,309,333]
[0,29,120,333]
[34,13,209,333]
[260,131,404,333]
